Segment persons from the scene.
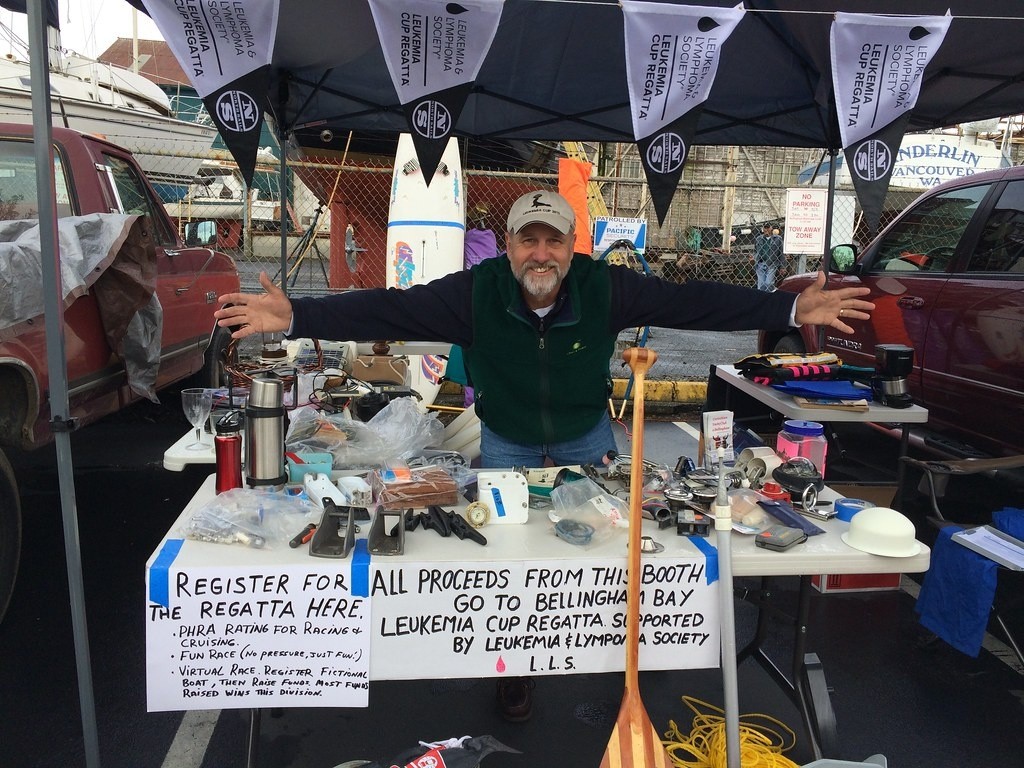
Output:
[755,222,787,292]
[214,192,876,724]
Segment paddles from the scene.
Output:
[600,346,674,768]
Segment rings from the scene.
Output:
[840,309,843,316]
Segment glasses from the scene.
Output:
[764,225,770,228]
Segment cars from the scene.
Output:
[0,124,241,631]
[759,171,1024,518]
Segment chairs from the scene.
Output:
[898,455,1024,667]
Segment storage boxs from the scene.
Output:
[353,355,409,384]
[811,486,902,593]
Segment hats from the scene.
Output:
[507,190,575,235]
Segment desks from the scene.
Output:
[163,407,351,471]
[705,364,931,487]
[144,468,931,768]
